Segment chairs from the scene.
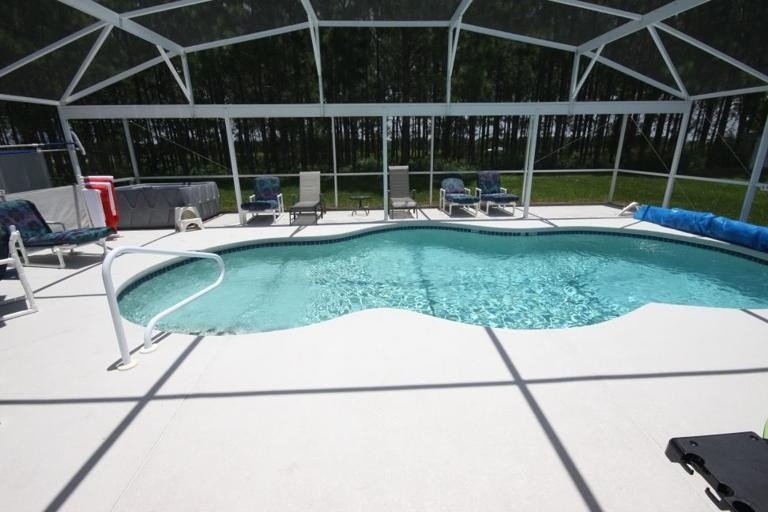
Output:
[0,197,118,322]
[239,171,325,224]
[386,165,518,220]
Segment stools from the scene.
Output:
[174,205,204,233]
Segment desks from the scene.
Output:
[348,194,372,216]
